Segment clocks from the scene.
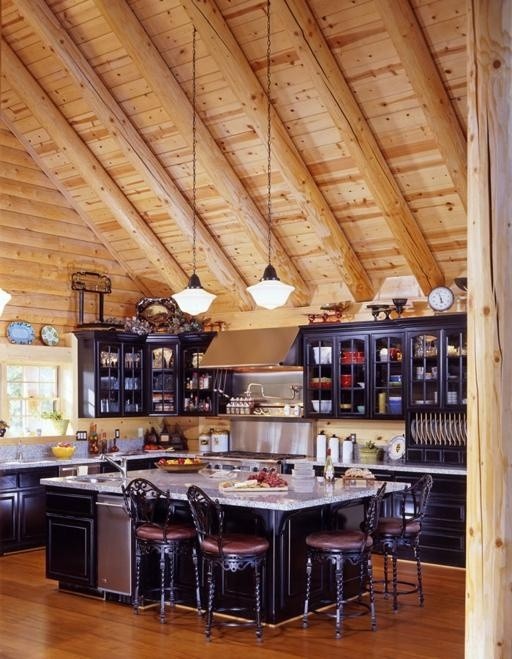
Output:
[428,285,455,312]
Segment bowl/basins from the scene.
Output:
[311,399,332,414]
[52,446,75,460]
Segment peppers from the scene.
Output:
[158,457,200,465]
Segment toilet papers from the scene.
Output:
[328,438,339,463]
[343,441,353,465]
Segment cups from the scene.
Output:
[341,374,352,387]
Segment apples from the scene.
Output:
[58,441,71,447]
[145,445,163,450]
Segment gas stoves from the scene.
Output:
[197,450,306,475]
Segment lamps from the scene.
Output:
[170,28,217,317]
[247,0,294,311]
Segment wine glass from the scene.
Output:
[100,350,141,368]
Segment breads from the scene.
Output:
[221,479,257,488]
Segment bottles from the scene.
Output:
[323,448,335,482]
[89,424,99,455]
[186,372,210,390]
[328,434,339,464]
[111,438,119,452]
[343,437,353,465]
[101,432,108,454]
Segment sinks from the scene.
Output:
[67,475,127,485]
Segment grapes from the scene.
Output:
[248,470,289,488]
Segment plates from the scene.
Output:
[6,321,34,345]
[387,434,406,461]
[154,460,209,473]
[40,325,59,347]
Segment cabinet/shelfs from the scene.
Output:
[72,330,232,418]
[299,314,466,421]
[1,466,59,557]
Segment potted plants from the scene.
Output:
[41,410,70,435]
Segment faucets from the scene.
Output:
[16,440,23,460]
[99,454,127,477]
